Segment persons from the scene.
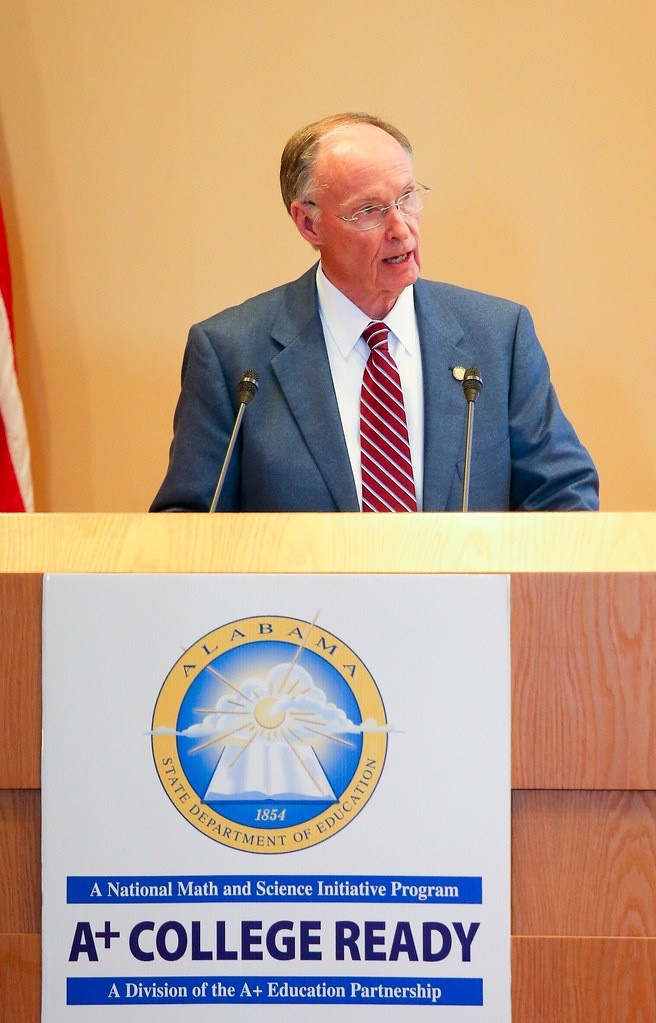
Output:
[147,113,599,512]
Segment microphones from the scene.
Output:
[462,367,483,512]
[210,369,259,511]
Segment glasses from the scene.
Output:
[301,179,431,231]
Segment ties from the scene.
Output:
[360,318,419,514]
